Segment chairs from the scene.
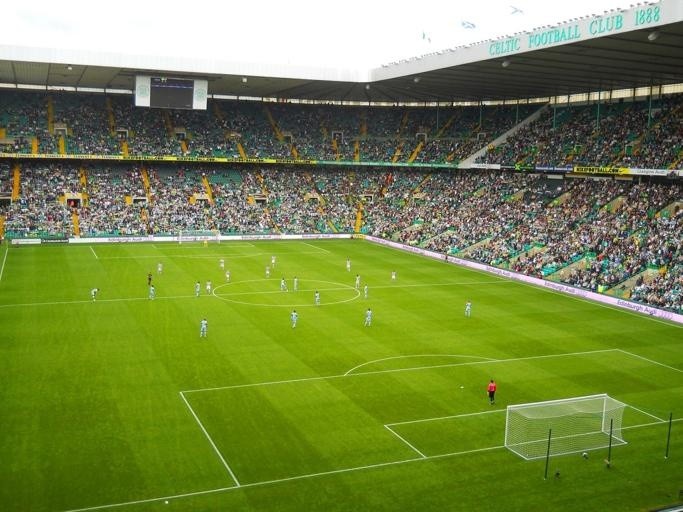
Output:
[0,85,682,324]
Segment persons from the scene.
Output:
[464,301,472,317]
[0,90,682,313]
[364,309,372,327]
[487,379,497,404]
[290,309,297,329]
[199,318,208,338]
